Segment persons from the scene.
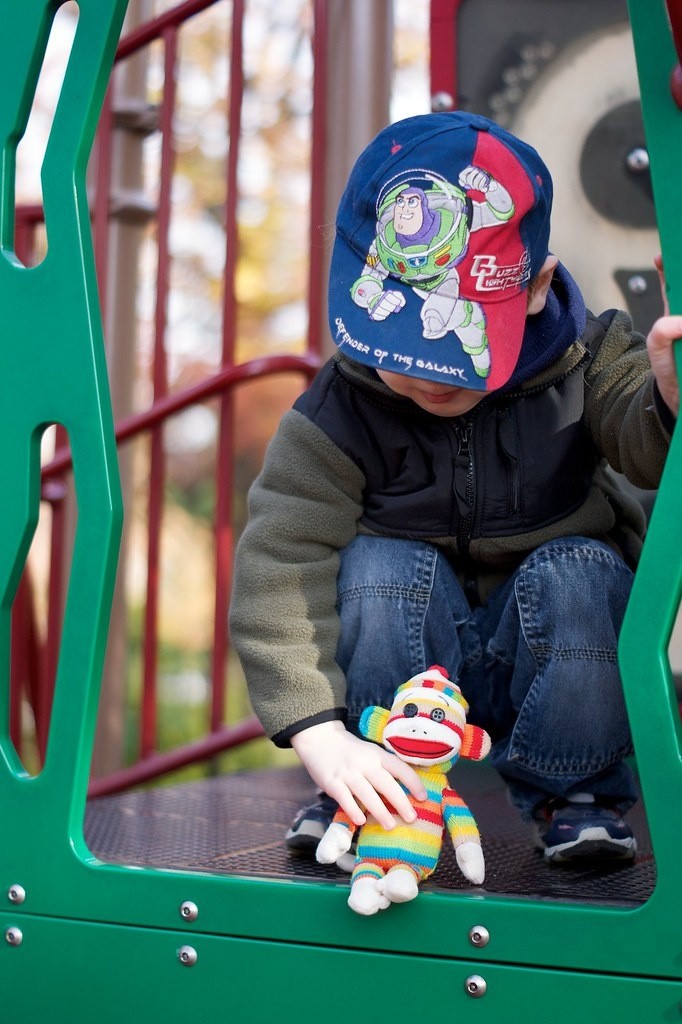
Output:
[227,111,682,859]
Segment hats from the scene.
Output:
[329,110,554,392]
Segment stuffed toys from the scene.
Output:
[315,665,489,915]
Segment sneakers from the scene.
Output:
[531,798,636,866]
[287,780,359,859]
